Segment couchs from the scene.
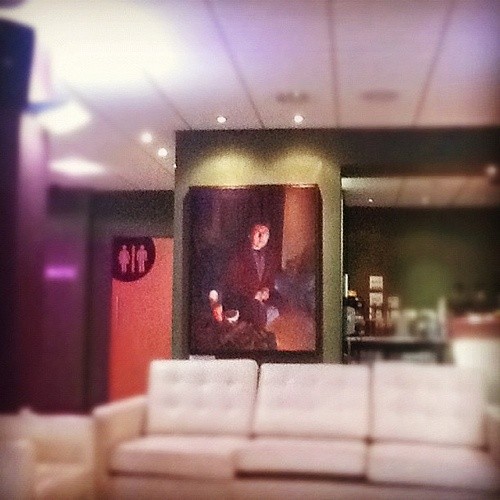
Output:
[0,410,97,500]
[90,356,500,500]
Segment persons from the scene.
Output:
[207,217,286,331]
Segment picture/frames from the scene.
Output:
[186,185,327,357]
